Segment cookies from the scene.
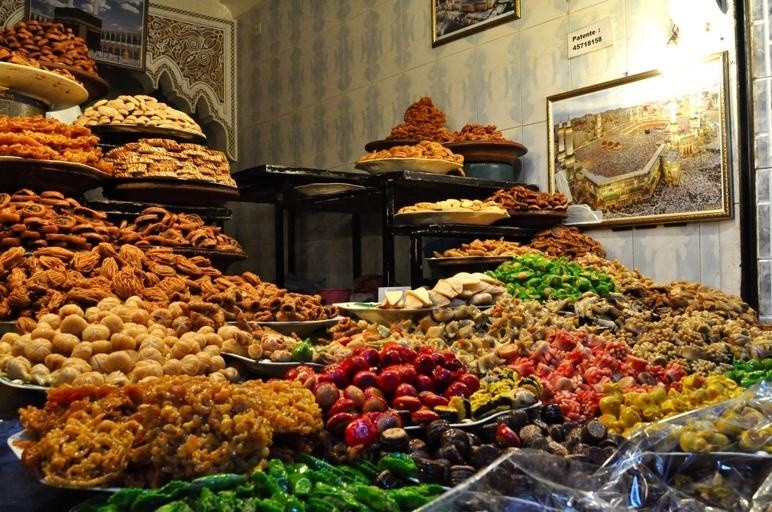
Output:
[73,95,201,132]
[0,189,243,252]
[0,114,112,172]
[106,138,237,186]
[0,20,98,86]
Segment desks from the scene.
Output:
[220,163,536,295]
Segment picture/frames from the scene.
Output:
[431,1,522,47]
[544,48,733,231]
[23,0,148,70]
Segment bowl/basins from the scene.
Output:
[445,140,527,164]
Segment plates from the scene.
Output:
[354,158,463,176]
[332,301,440,323]
[505,209,566,226]
[426,256,512,264]
[401,400,543,432]
[6,429,159,495]
[0,154,113,181]
[222,315,346,337]
[394,210,512,225]
[293,182,365,199]
[0,61,89,112]
[0,371,54,400]
[0,318,20,332]
[220,350,325,373]
[84,122,207,144]
[102,177,239,192]
[362,140,418,152]
